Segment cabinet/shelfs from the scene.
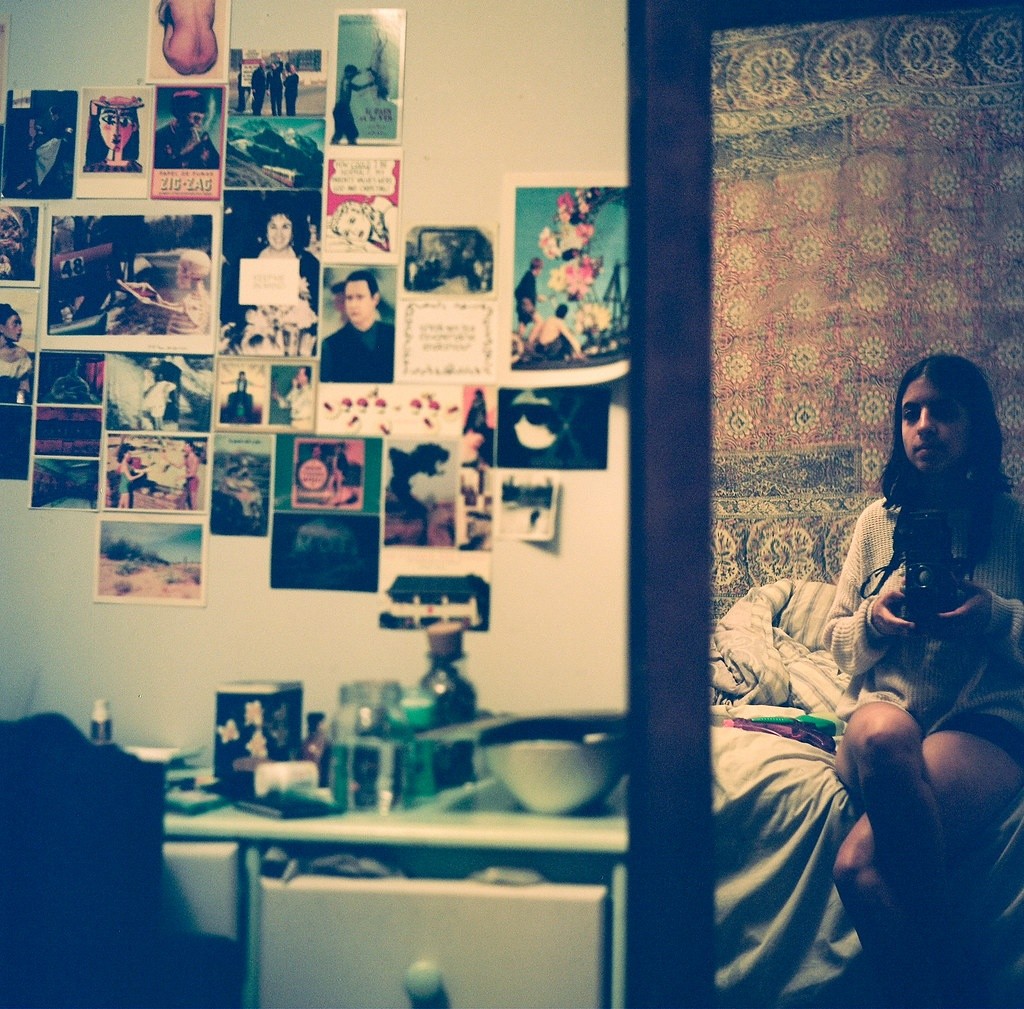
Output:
[161,774,629,1009]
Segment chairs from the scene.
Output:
[0,712,246,1009]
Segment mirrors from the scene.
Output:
[623,0,1024,1009]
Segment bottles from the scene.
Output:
[300,620,476,814]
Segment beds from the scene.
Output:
[708,574,1024,1009]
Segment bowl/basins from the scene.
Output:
[479,715,624,815]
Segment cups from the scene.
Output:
[215,682,303,773]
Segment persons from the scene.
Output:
[223,201,319,356]
[152,91,220,170]
[272,366,313,428]
[320,271,395,384]
[116,443,152,507]
[161,440,199,509]
[159,0,218,75]
[511,257,584,365]
[408,231,493,292]
[329,197,396,253]
[0,303,34,404]
[822,354,1024,1009]
[236,54,299,116]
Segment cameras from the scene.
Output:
[903,509,974,629]
